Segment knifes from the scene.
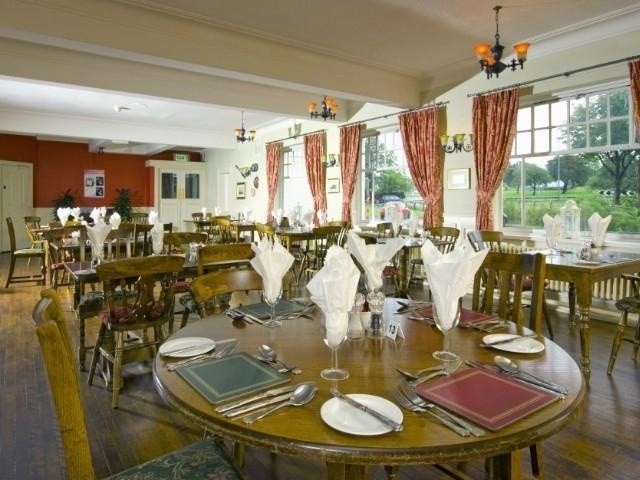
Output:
[479,334,539,349]
[225,387,320,418]
[215,385,296,413]
[226,308,255,325]
[159,338,237,359]
[330,388,404,432]
[234,309,264,324]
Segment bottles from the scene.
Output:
[189,242,199,266]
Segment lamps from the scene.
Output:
[234,110,256,144]
[439,133,473,154]
[309,95,339,121]
[321,153,336,168]
[472,5,531,80]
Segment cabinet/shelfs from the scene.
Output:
[144,159,207,233]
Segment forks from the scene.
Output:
[482,322,511,332]
[397,382,485,437]
[467,320,506,328]
[390,386,471,436]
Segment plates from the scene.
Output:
[320,393,406,437]
[482,334,545,356]
[158,336,215,359]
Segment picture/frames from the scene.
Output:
[328,178,340,193]
[447,167,471,190]
[236,182,246,198]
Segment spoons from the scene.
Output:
[167,341,241,374]
[493,355,570,396]
[226,312,246,320]
[242,383,315,426]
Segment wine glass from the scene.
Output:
[258,274,462,381]
[546,235,605,260]
